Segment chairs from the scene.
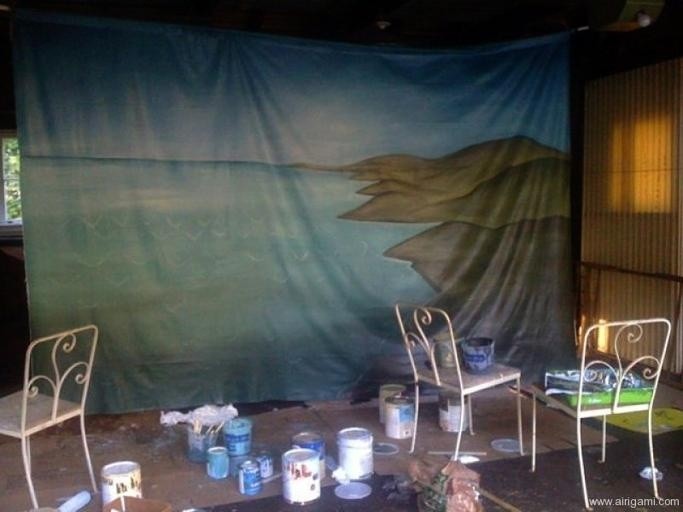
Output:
[523,313,674,512]
[0,321,103,511]
[391,299,525,465]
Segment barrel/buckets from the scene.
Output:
[432,331,465,369]
[437,396,470,435]
[336,426,375,481]
[280,448,322,507]
[291,430,328,479]
[100,460,143,505]
[461,336,496,376]
[378,383,407,424]
[383,394,416,441]
